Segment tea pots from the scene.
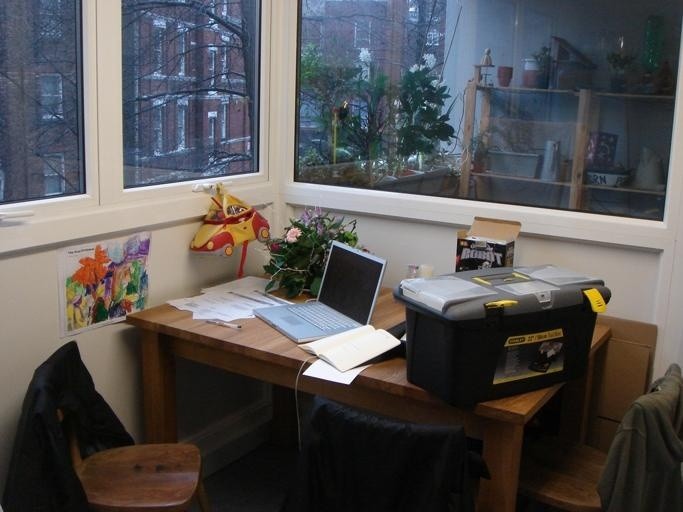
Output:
[630,146,666,192]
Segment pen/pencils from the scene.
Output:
[206,320,241,329]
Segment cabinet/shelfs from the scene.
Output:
[460,66,676,222]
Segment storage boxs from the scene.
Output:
[390,264,612,407]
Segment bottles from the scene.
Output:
[539,139,562,182]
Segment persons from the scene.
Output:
[67,293,133,327]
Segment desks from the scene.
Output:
[123,273,612,512]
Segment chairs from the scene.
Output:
[515,362,682,512]
[303,391,467,511]
[31,340,210,511]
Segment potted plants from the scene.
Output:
[303,53,458,194]
[470,104,544,178]
[605,48,631,93]
[532,47,555,90]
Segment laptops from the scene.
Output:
[253,239,388,344]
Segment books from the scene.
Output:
[297,323,402,372]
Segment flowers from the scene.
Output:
[260,203,358,299]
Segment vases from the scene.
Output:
[496,58,539,89]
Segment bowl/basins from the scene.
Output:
[583,171,632,187]
